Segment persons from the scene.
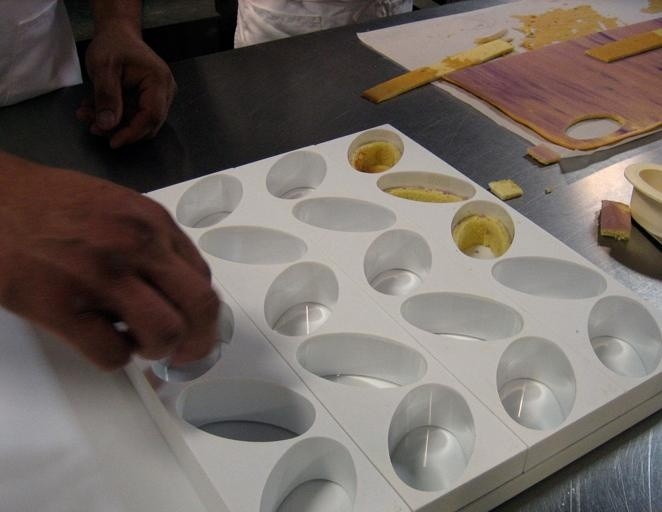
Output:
[0,1,221,376]
